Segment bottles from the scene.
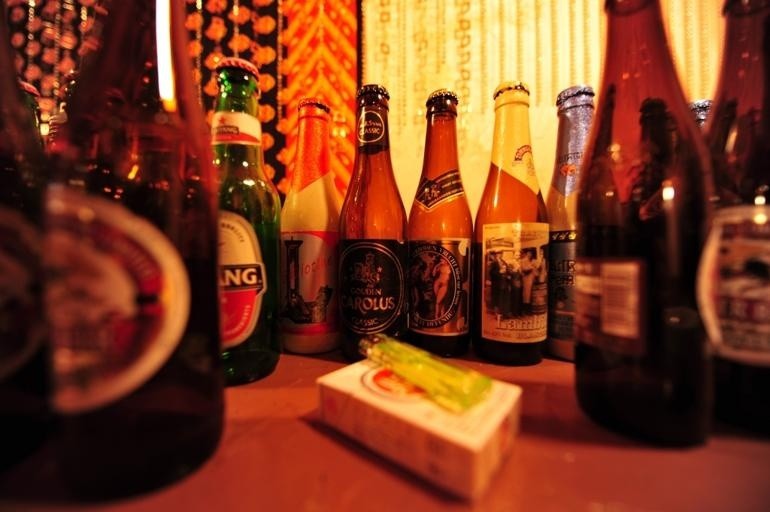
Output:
[278,93,335,357]
[542,85,598,361]
[571,1,714,447]
[402,88,476,359]
[1,48,224,510]
[467,81,551,363]
[206,55,279,388]
[699,1,769,441]
[688,96,715,126]
[327,84,403,363]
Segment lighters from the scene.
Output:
[357,331,491,415]
